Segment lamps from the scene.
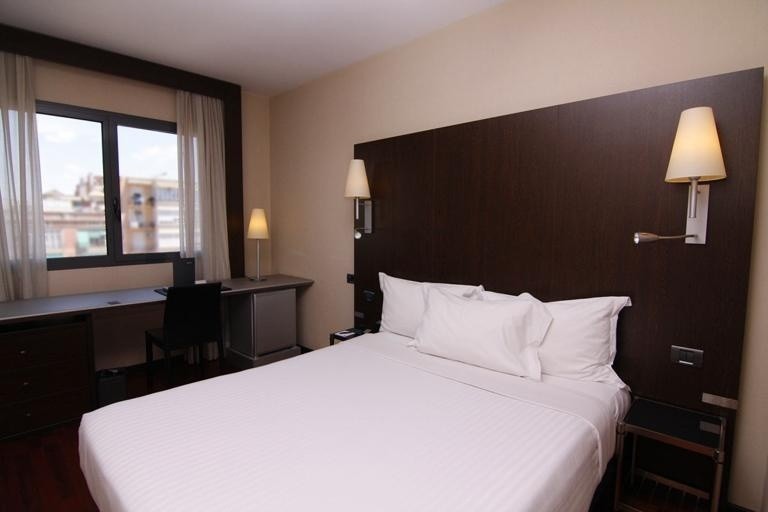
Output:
[633,104,729,249]
[245,207,271,282]
[340,158,375,242]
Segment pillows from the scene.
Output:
[376,268,634,390]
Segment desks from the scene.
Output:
[0,274,312,443]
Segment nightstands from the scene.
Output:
[613,398,728,512]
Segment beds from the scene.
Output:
[76,334,632,512]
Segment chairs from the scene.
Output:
[145,282,225,386]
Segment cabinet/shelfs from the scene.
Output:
[0,322,93,441]
